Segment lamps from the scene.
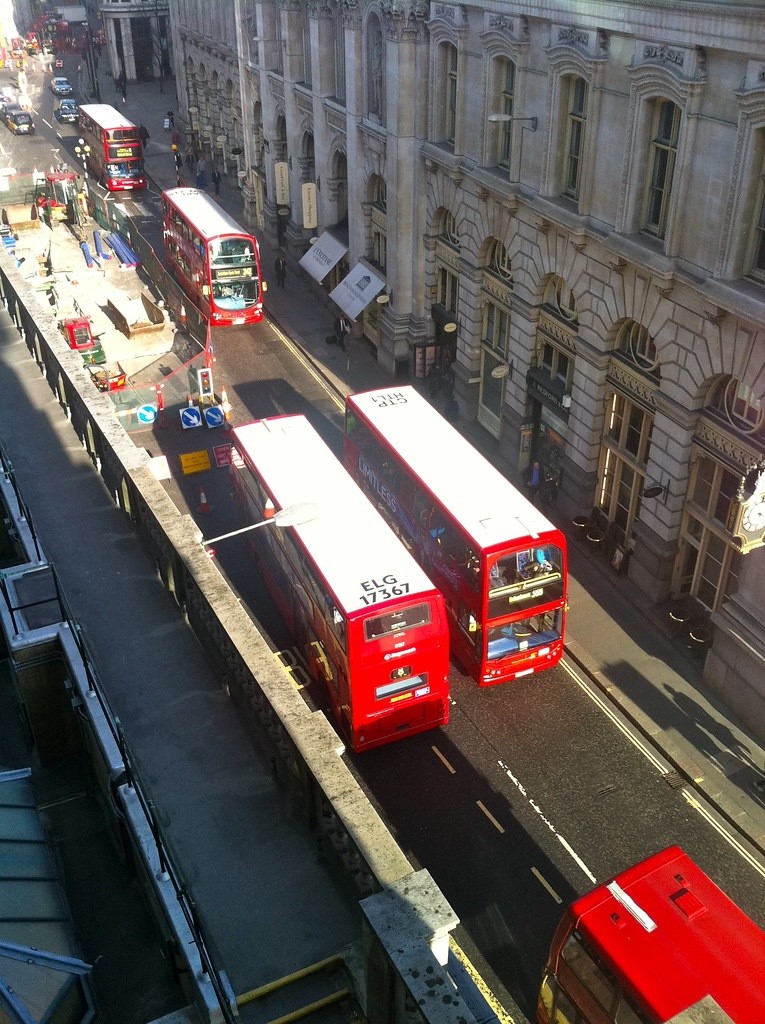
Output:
[253,36,286,50]
[486,114,539,132]
[561,394,572,407]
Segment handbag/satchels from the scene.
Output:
[123,98,125,103]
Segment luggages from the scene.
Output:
[326,329,337,344]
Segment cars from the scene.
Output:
[60,99,78,124]
[51,77,72,94]
[1,102,22,114]
[6,110,35,136]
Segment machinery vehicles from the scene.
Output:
[34,172,84,221]
[57,318,107,367]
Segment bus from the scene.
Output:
[77,103,144,191]
[534,843,765,1024]
[162,187,266,327]
[343,383,569,689]
[228,411,449,753]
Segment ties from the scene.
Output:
[342,320,345,330]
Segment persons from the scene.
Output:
[446,395,459,420]
[186,150,193,169]
[167,108,174,127]
[535,545,551,568]
[197,172,207,189]
[121,81,126,97]
[524,461,542,502]
[429,363,441,397]
[140,124,149,149]
[334,314,352,350]
[94,56,98,68]
[198,156,206,173]
[275,255,286,288]
[212,168,221,195]
[173,131,179,145]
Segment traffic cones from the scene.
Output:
[221,387,232,421]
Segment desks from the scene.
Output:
[587,530,607,557]
[572,516,590,542]
[669,609,691,636]
[688,627,711,664]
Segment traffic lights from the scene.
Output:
[198,368,213,396]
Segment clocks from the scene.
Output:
[743,497,765,534]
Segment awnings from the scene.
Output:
[328,262,385,322]
[298,231,348,285]
